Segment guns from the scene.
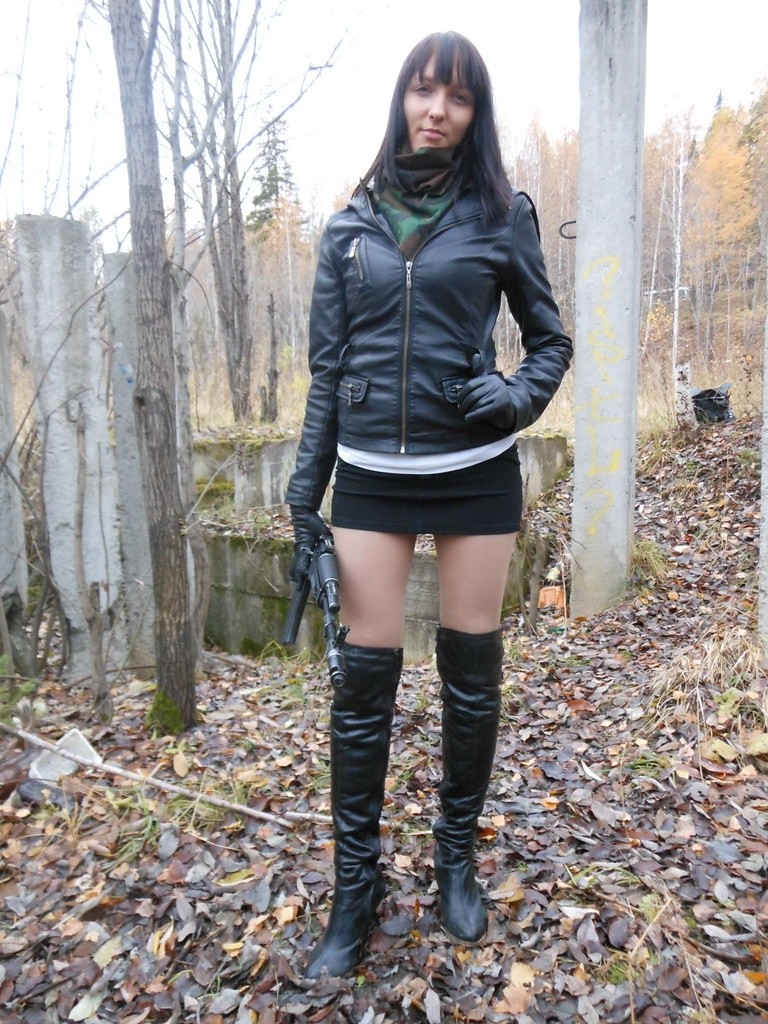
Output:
[281,516,353,690]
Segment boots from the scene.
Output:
[433,624,503,948]
[303,641,404,979]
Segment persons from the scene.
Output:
[282,29,577,988]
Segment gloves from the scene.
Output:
[287,503,334,592]
[455,372,533,439]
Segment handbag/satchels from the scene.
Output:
[692,388,736,423]
